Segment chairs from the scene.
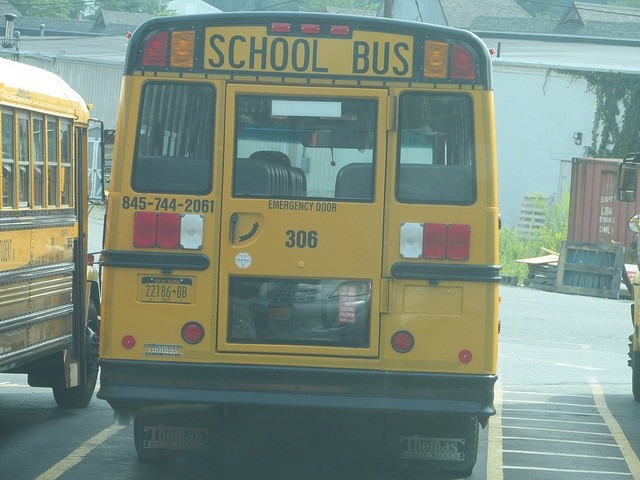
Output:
[335,163,474,203]
[130,152,307,197]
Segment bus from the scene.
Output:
[96,9,502,479]
[616,152,640,400]
[0,53,106,408]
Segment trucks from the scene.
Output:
[566,157,640,265]
